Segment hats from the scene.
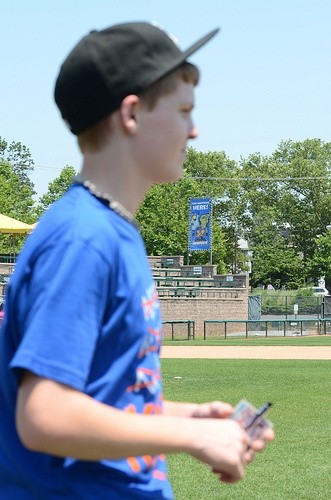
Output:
[52,20,220,136]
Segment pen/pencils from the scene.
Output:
[245,402,272,430]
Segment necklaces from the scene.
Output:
[77,173,141,228]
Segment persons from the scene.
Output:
[0,21,276,500]
